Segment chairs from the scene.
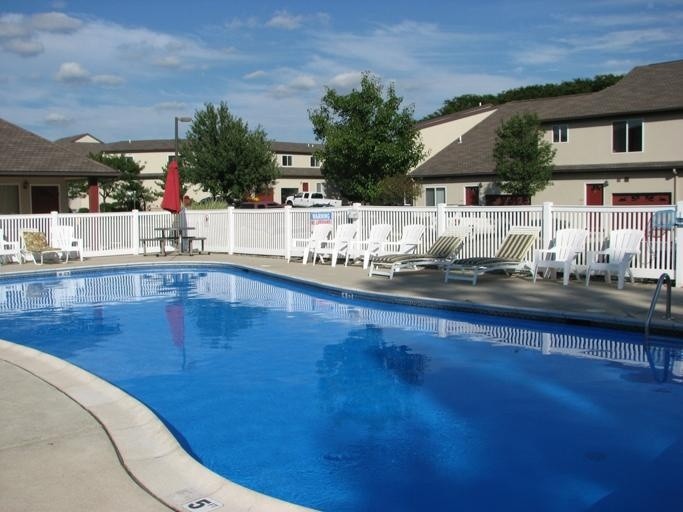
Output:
[0,224,85,262]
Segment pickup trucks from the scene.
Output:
[285,191,343,208]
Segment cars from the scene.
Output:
[428,202,510,238]
[198,194,237,209]
[234,200,284,209]
[636,209,676,262]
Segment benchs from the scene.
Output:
[178,237,206,255]
[141,237,166,257]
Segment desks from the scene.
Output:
[153,225,195,253]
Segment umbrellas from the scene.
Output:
[159,161,181,256]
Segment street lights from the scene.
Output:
[173,115,192,162]
